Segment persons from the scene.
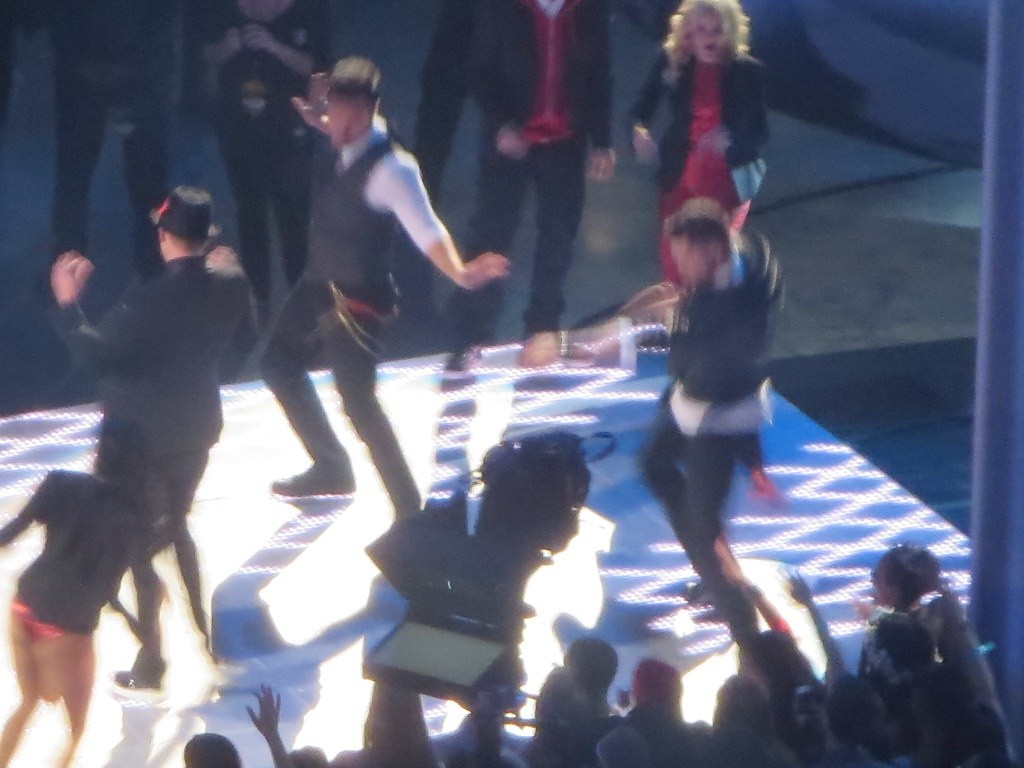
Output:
[627,0,769,288]
[638,196,784,626]
[48,185,252,689]
[1,421,164,768]
[184,683,525,768]
[39,0,178,304]
[261,56,511,522]
[525,544,1017,768]
[199,0,332,329]
[383,0,615,349]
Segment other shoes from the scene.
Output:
[116,663,166,690]
[749,471,780,502]
[682,581,717,608]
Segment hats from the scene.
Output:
[148,186,224,240]
[633,658,680,701]
[597,726,650,768]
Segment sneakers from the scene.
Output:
[443,344,484,381]
[272,463,358,496]
